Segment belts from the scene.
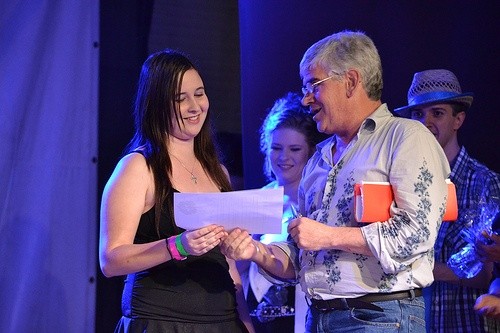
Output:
[311,288,424,308]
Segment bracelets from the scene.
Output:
[165,233,189,261]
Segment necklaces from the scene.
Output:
[167,149,199,185]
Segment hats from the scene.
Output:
[395,70,474,117]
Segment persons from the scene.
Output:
[218,32,452,333]
[393,69,500,333]
[243,93,322,333]
[98,51,255,333]
[471,230,500,320]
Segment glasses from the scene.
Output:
[302,74,337,95]
